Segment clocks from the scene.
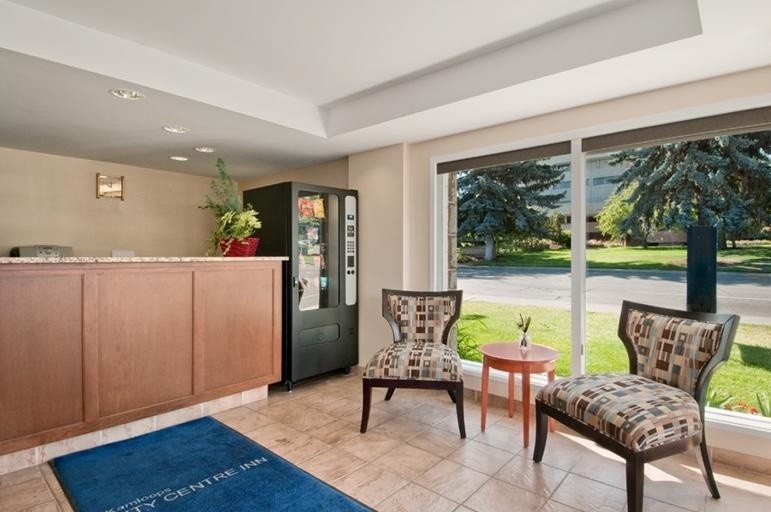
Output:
[96,173,125,200]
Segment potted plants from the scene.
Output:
[198,157,261,256]
[518,312,531,349]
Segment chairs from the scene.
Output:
[361,289,466,440]
[534,301,739,512]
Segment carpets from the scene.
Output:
[38,417,379,512]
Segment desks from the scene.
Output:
[477,342,558,447]
